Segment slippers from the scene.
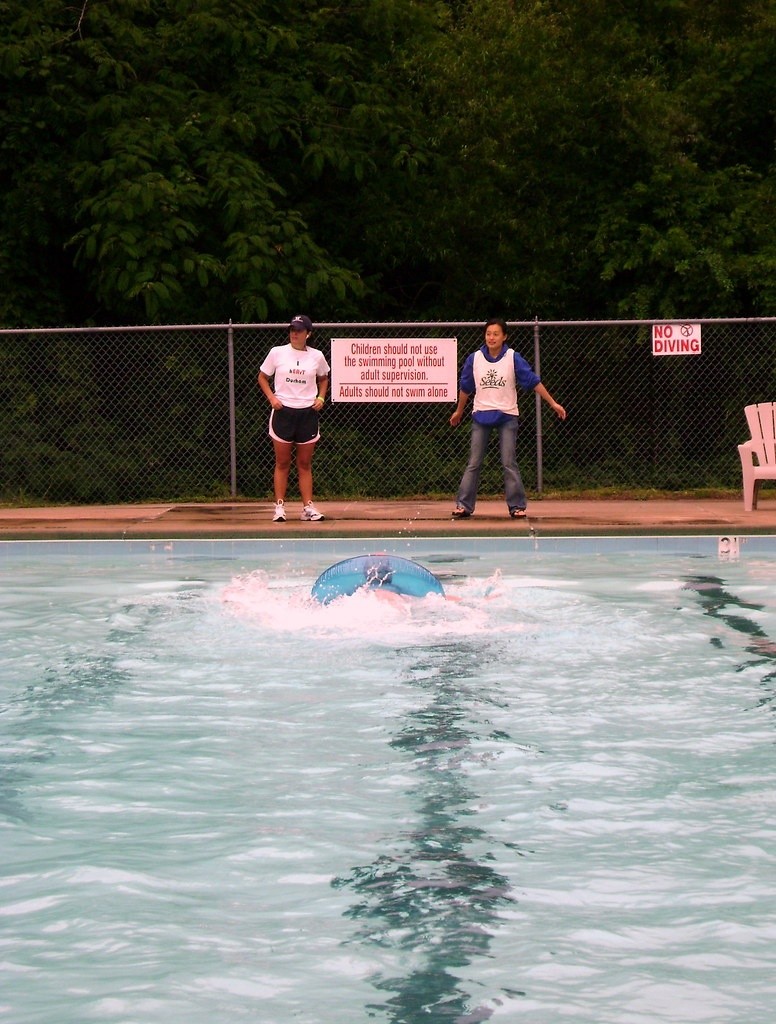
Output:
[452,508,470,516]
[513,509,526,518]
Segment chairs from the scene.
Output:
[737,402,776,512]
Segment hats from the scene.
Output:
[286,315,312,330]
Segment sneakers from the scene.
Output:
[273,499,286,522]
[301,500,324,521]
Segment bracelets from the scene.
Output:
[317,396,325,403]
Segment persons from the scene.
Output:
[257,315,330,523]
[450,319,566,519]
[363,552,393,584]
